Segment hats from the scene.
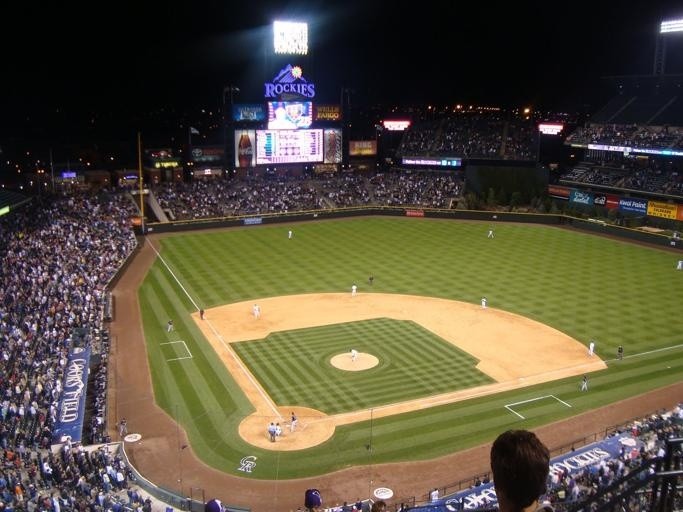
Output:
[303,488,323,509]
[203,498,234,511]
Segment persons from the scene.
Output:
[676,259,682,269]
[274,422,280,436]
[669,230,677,248]
[480,296,487,310]
[351,283,357,295]
[268,422,275,442]
[137,96,683,224]
[487,225,494,239]
[580,375,588,392]
[368,274,373,285]
[290,411,296,433]
[199,306,203,319]
[253,303,259,318]
[427,401,683,511]
[617,344,623,360]
[0,158,138,511]
[167,319,173,333]
[287,229,292,240]
[349,348,358,361]
[588,340,594,354]
[138,484,426,512]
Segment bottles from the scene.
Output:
[238,130,253,168]
